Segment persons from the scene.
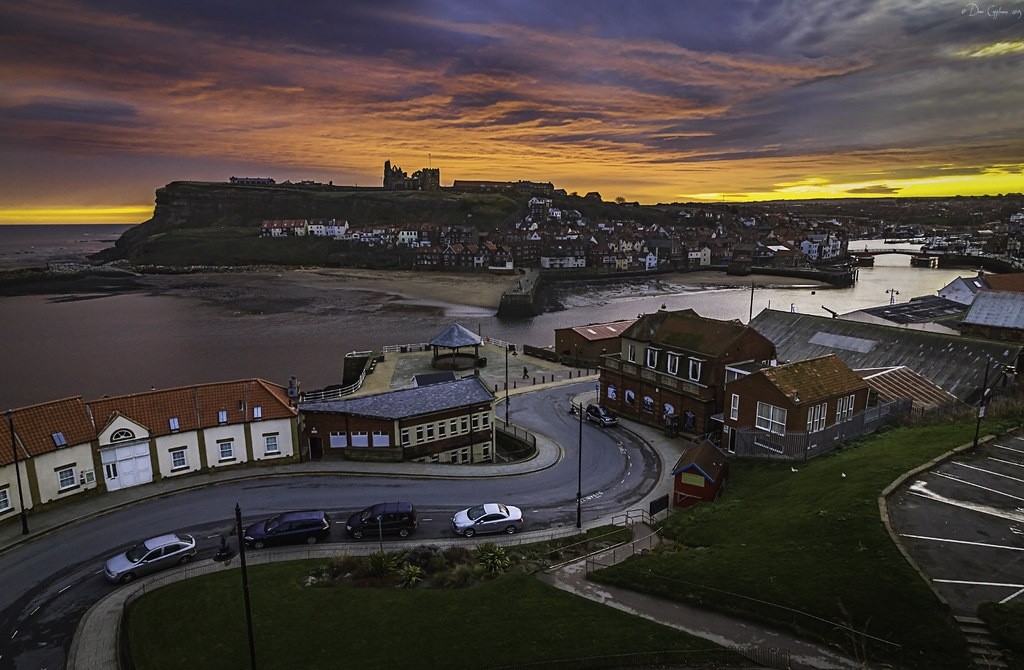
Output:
[523,366,530,380]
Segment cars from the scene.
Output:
[452,503,524,538]
[345,502,419,539]
[244,510,331,550]
[585,403,619,428]
[103,533,198,586]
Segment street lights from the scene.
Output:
[973,356,1018,449]
[505,345,519,426]
[567,401,583,528]
[886,288,899,305]
[213,502,256,670]
[5,410,31,535]
